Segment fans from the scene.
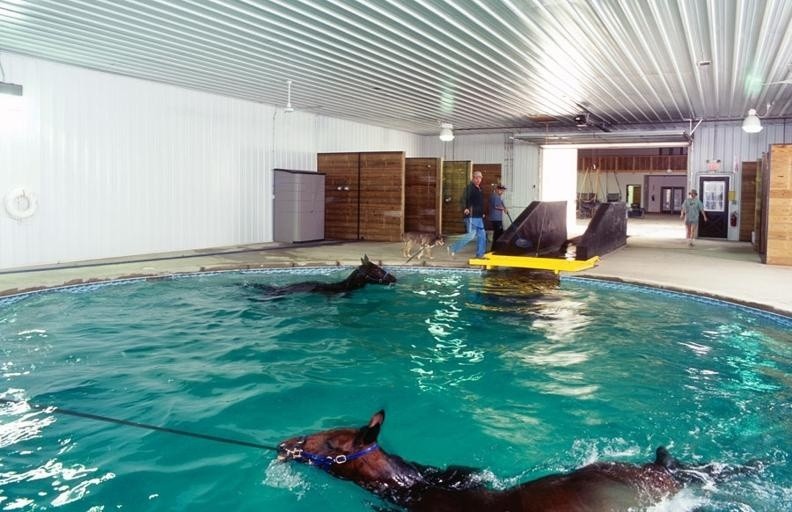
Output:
[257,79,323,114]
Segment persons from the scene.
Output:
[445,170,487,259]
[679,189,708,248]
[488,183,510,252]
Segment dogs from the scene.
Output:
[401,230,446,261]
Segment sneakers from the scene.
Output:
[447,246,455,262]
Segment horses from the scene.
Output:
[274,406,765,512]
[228,253,398,329]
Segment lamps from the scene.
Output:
[741,109,763,134]
[439,128,454,142]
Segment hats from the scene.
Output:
[689,189,697,195]
[473,171,483,178]
[497,184,505,190]
